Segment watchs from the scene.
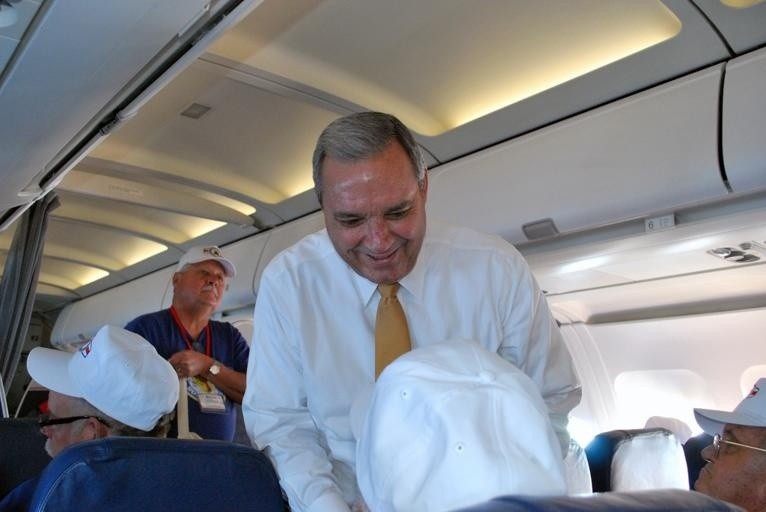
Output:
[206,360,222,381]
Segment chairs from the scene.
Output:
[31,440,285,512]
[455,488,746,512]
[682,429,713,490]
[584,428,683,492]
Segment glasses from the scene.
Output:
[712,433,765,457]
[38,416,111,433]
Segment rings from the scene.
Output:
[177,367,181,373]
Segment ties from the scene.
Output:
[374,283,411,380]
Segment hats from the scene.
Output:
[693,377,766,436]
[27,324,181,432]
[178,245,236,278]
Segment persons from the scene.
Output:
[692,377,766,512]
[241,111,583,512]
[124,244,249,442]
[0,323,180,511]
